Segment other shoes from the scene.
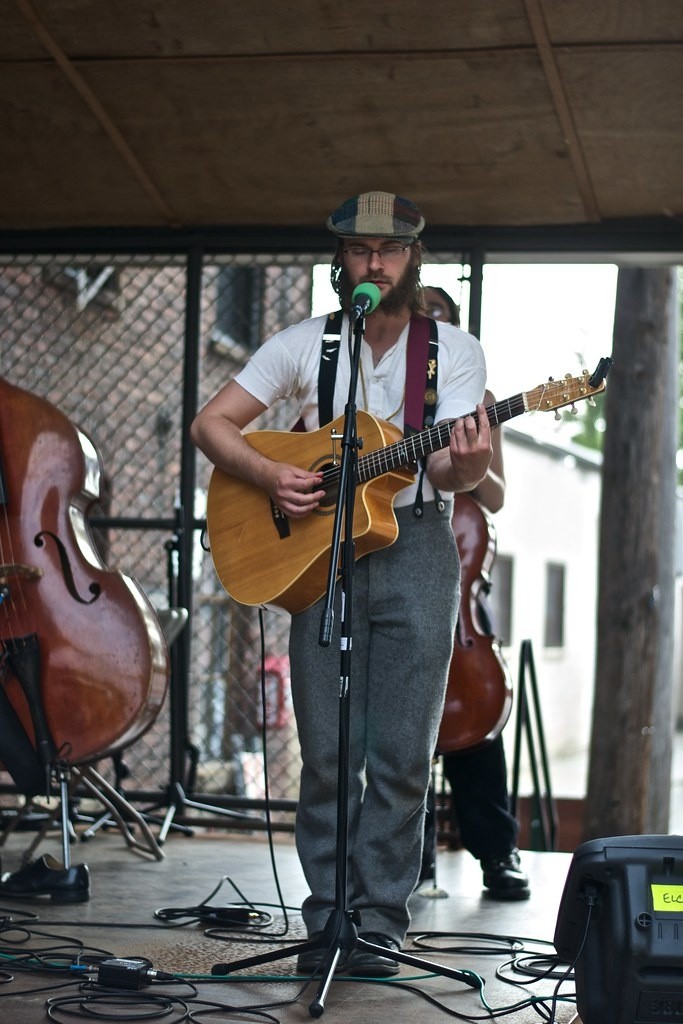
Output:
[479,850,533,901]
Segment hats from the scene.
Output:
[326,190,425,244]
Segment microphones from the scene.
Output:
[352,283,381,320]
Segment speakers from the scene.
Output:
[553,835,683,1024]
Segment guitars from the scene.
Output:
[202,368,608,616]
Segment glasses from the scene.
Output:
[342,245,409,261]
[424,307,446,321]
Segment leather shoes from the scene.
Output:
[296,932,402,977]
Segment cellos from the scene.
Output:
[431,494,515,890]
[0,375,173,868]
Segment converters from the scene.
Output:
[201,905,249,926]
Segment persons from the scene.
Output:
[417,286,531,900]
[191,192,492,977]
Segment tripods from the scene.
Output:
[212,317,485,1015]
[0,542,266,860]
